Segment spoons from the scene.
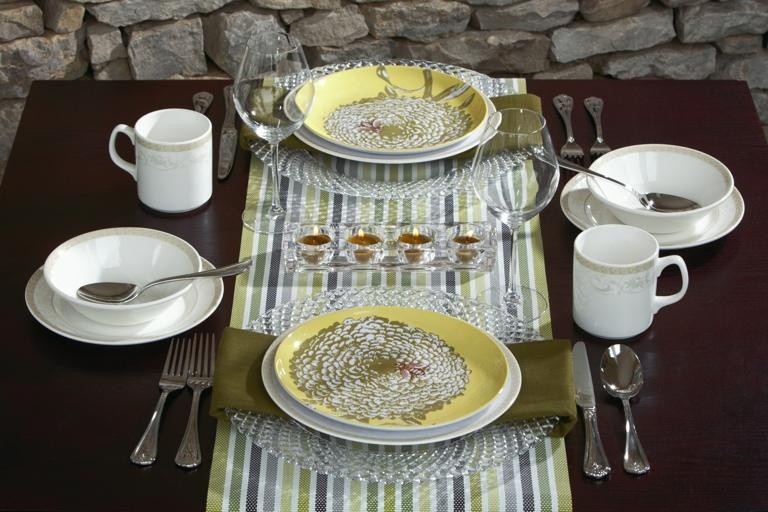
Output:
[528,144,702,212]
[602,338,653,476]
[77,258,253,305]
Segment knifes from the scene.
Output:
[569,342,609,483]
[219,82,238,180]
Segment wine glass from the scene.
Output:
[466,108,562,322]
[230,32,314,237]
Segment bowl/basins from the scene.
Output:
[589,144,735,227]
[41,227,201,327]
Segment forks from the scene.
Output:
[584,95,610,164]
[550,91,583,163]
[175,332,218,469]
[129,332,191,468]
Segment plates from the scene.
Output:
[21,249,224,346]
[283,64,500,165]
[258,307,524,448]
[560,160,748,251]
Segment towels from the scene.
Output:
[212,326,578,445]
[240,85,544,151]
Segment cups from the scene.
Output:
[571,223,691,343]
[105,107,215,214]
[293,223,488,267]
